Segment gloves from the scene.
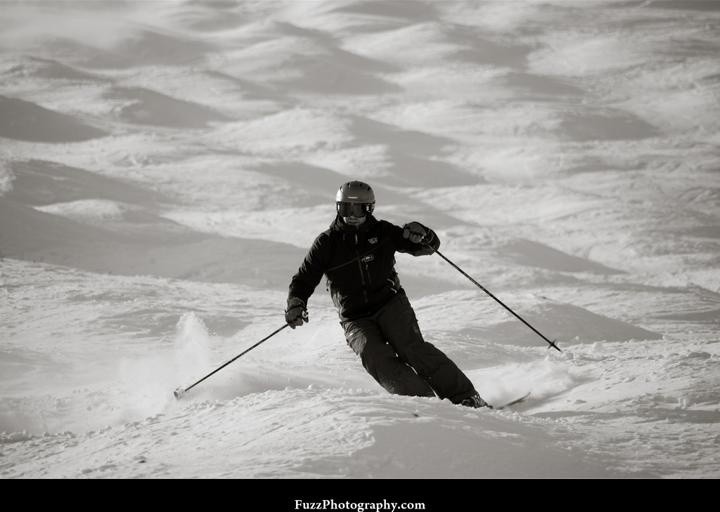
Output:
[402,220,427,243]
[285,298,309,329]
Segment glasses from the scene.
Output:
[337,205,369,218]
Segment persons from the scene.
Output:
[284,180,495,409]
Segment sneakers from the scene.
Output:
[461,393,488,407]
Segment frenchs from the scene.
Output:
[495,391,532,410]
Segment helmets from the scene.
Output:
[335,180,375,218]
